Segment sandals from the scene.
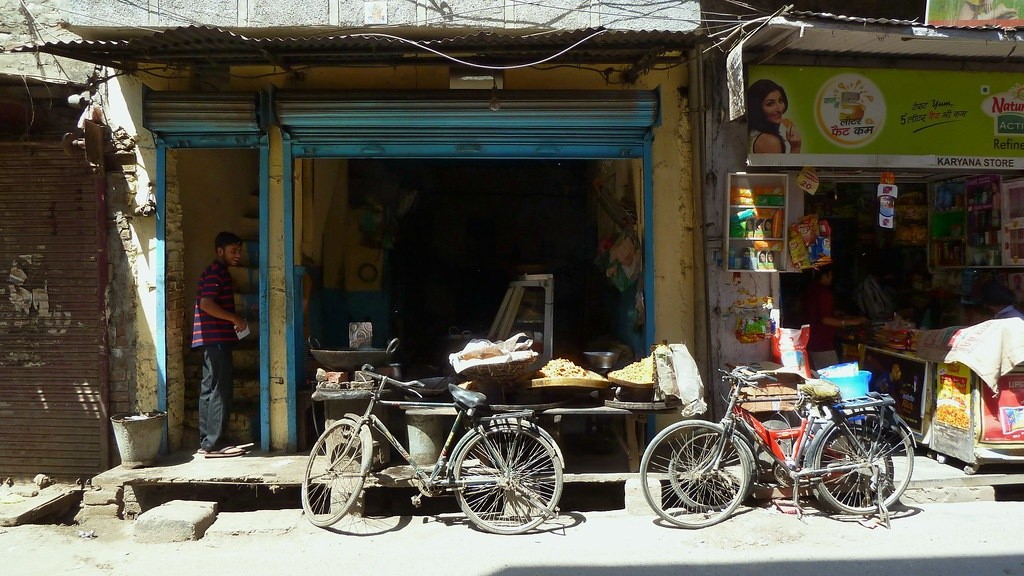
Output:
[205,446,246,457]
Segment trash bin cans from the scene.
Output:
[110,410,167,468]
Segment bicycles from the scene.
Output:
[636,366,916,531]
[298,362,566,535]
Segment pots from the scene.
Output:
[308,336,399,371]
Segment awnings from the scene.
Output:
[13,28,709,66]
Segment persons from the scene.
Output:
[758,251,775,269]
[745,218,772,238]
[799,265,867,370]
[749,79,801,155]
[191,231,247,457]
[983,283,1023,319]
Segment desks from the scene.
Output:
[399,395,676,473]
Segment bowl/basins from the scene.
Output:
[583,351,619,368]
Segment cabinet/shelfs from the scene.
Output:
[722,173,789,272]
[927,174,1004,273]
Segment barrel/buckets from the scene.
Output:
[819,368,872,421]
[110,410,167,467]
